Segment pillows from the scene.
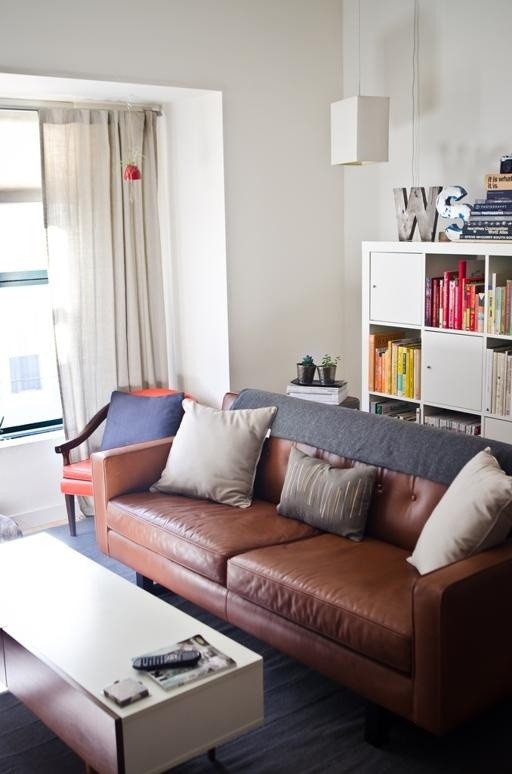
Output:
[275,448,378,543]
[101,390,185,450]
[404,445,512,577]
[148,397,278,509]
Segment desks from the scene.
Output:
[340,396,360,411]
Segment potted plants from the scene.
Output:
[296,355,317,385]
[316,353,341,385]
[119,150,145,181]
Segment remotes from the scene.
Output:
[133,650,201,670]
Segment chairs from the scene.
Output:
[55,388,198,537]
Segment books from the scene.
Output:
[460,153,512,243]
[423,413,480,435]
[369,329,421,423]
[485,347,511,417]
[130,632,236,692]
[426,253,511,339]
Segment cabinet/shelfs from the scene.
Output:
[360,240,512,444]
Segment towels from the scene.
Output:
[226,388,512,483]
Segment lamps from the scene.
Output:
[329,0,389,166]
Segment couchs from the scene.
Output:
[88,391,512,734]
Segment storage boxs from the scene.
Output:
[287,382,348,405]
